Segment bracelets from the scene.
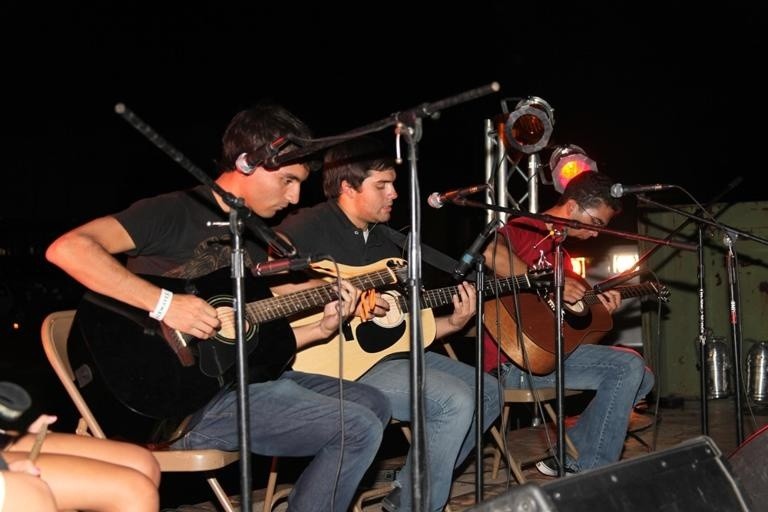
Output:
[149,286,173,322]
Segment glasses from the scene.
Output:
[577,200,604,226]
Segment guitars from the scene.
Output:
[483,271,671,374]
[66,260,410,443]
[269,259,553,382]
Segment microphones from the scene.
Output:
[233,134,289,176]
[609,181,667,201]
[427,183,493,212]
[250,258,309,281]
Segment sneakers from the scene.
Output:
[382,485,402,511]
[536,457,576,477]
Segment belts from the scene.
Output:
[490,364,509,378]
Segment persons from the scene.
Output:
[475,174,653,478]
[274,135,504,510]
[46,108,391,510]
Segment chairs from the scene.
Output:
[41,310,240,511]
[264,418,452,512]
[440,324,582,478]
